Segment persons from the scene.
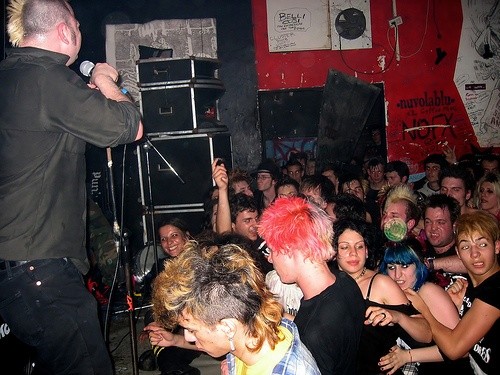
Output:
[0,0,145,375]
[137,152,500,375]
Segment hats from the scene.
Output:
[421,154,449,165]
[256,162,280,177]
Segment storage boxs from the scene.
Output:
[135,56,234,245]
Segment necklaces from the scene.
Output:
[354,267,366,280]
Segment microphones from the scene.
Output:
[80,61,131,98]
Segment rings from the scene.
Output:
[381,313,387,319]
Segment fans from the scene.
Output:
[329,0,373,49]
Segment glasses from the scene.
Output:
[257,241,269,257]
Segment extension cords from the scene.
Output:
[389,16,403,28]
